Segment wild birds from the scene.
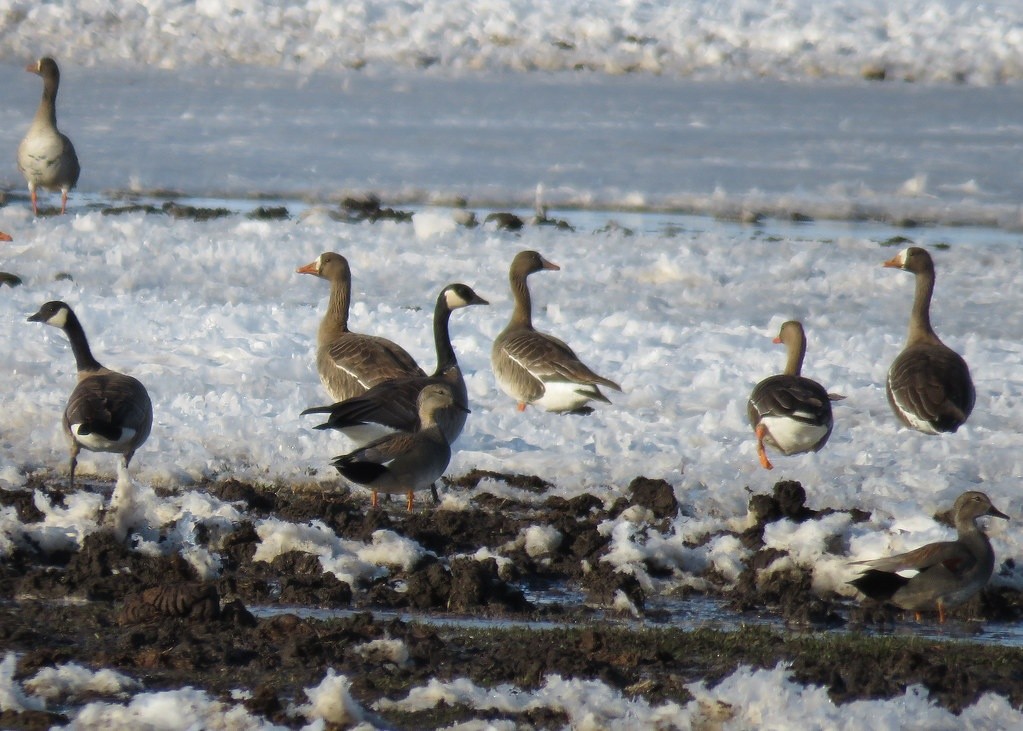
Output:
[844,491,1010,625]
[489,251,623,413]
[882,247,976,435]
[28,301,153,489]
[17,57,81,217]
[299,283,489,507]
[295,252,428,404]
[328,383,472,513]
[747,321,834,470]
